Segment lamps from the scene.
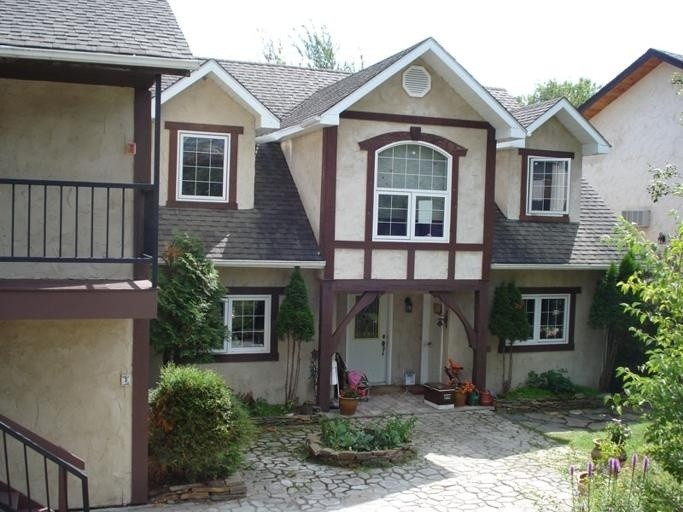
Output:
[436,316,448,328]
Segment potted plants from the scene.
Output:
[337,390,360,416]
